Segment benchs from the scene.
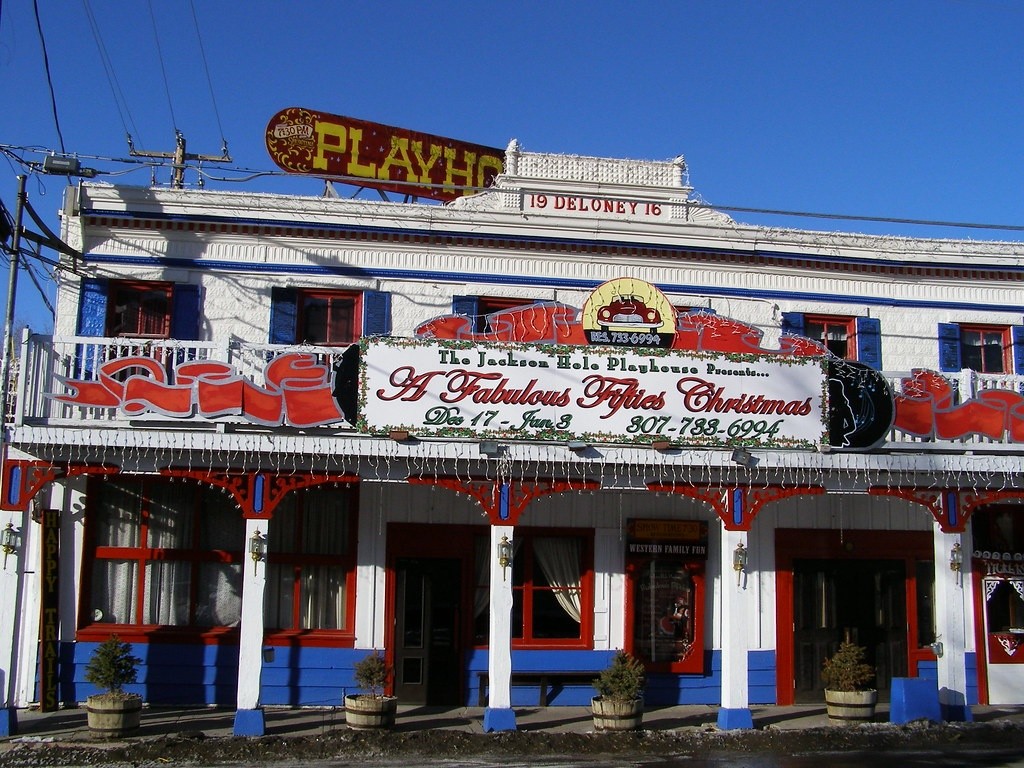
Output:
[477,671,604,708]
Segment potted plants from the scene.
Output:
[345,647,398,733]
[824,639,879,726]
[591,647,644,731]
[83,635,144,739]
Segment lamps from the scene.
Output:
[249,527,264,578]
[479,440,499,455]
[733,538,746,587]
[497,532,512,582]
[731,449,752,465]
[567,441,588,451]
[652,441,670,450]
[949,540,963,585]
[0,518,17,569]
